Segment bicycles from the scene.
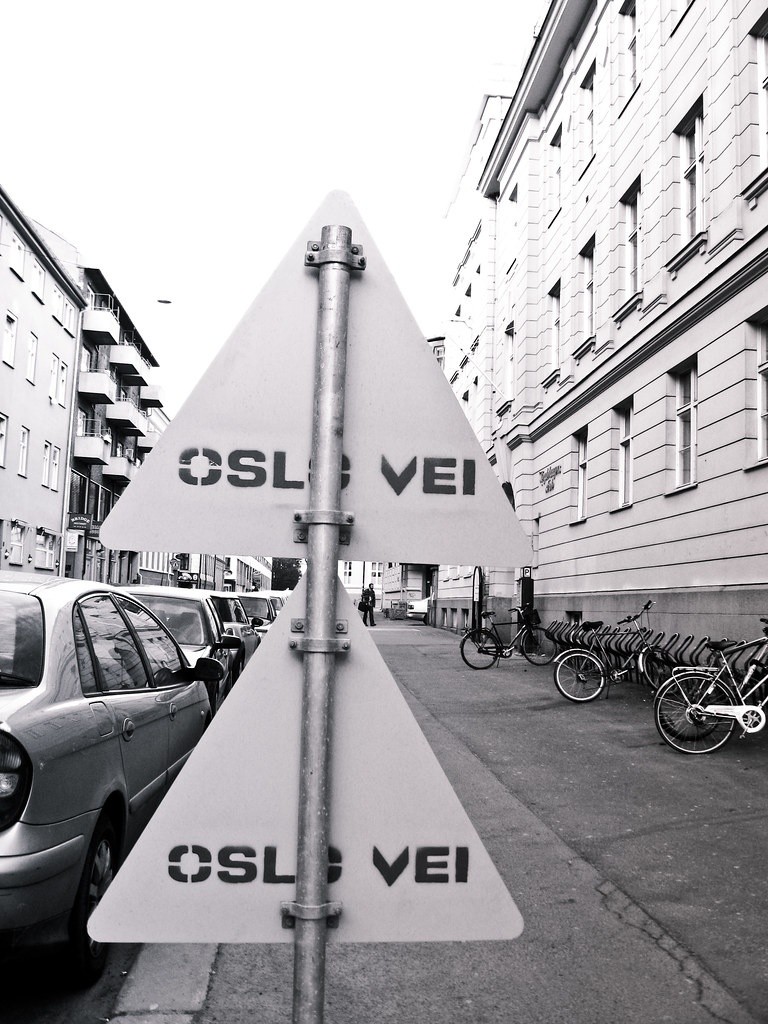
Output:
[460,602,559,671]
[554,599,682,704]
[653,616,768,755]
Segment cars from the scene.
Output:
[238,590,291,640]
[0,571,226,946]
[195,588,265,673]
[113,585,243,716]
[406,594,434,625]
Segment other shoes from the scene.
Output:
[366,624,368,626]
[370,623,376,626]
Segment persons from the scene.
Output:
[361,583,376,626]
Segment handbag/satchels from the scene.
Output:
[358,596,369,612]
